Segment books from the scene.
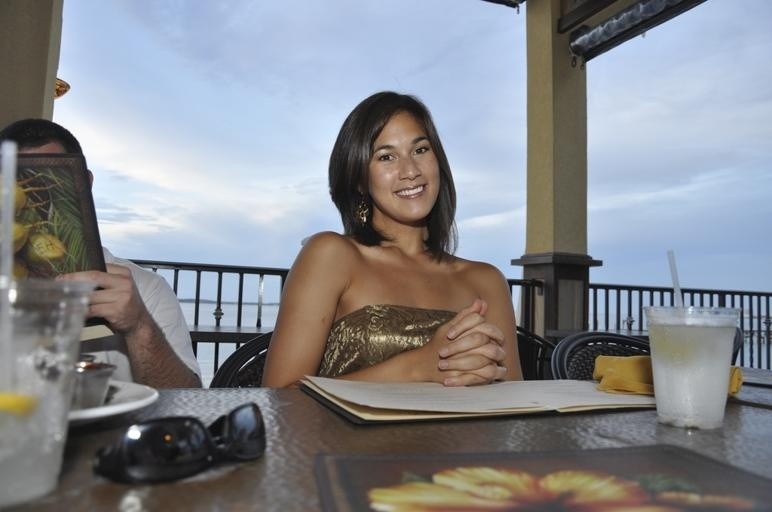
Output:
[13,152,116,348]
[296,374,656,428]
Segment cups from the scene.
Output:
[71,354,117,410]
[0,278,100,508]
[643,306,741,429]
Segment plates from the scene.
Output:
[68,379,160,426]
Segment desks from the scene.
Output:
[187,324,275,343]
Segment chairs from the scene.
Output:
[516,325,556,380]
[550,331,649,379]
[209,331,274,387]
[731,327,744,366]
[0,366,772,511]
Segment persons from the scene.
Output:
[259,91,524,388]
[1,117,204,390]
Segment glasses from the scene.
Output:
[93,402,267,486]
